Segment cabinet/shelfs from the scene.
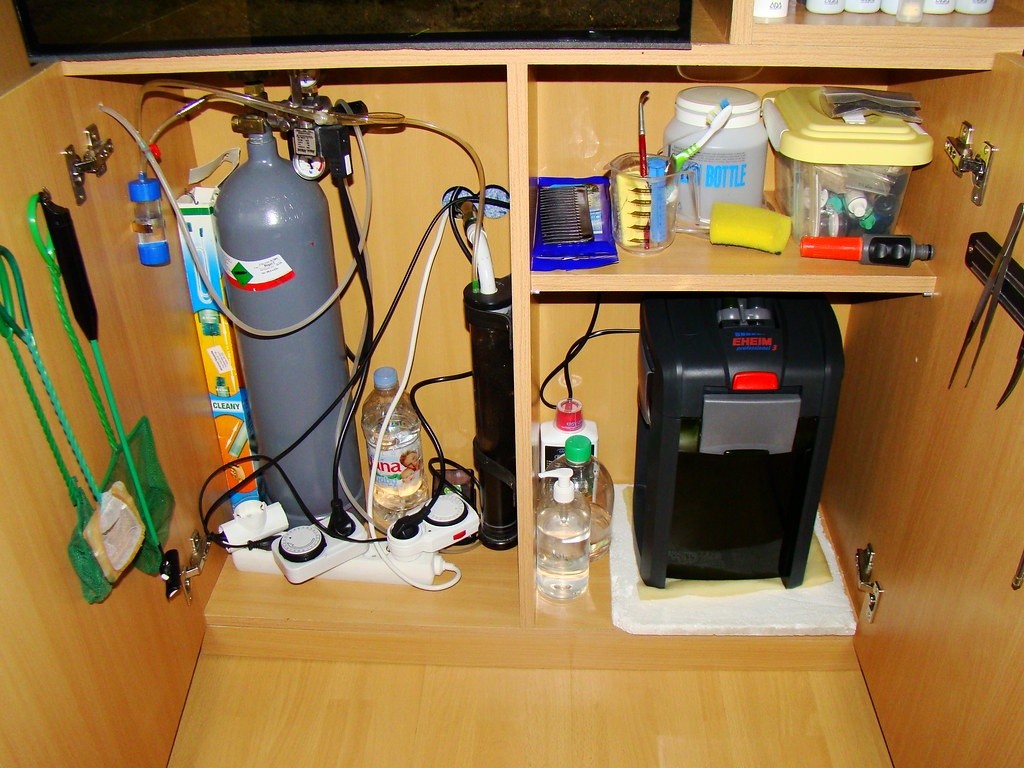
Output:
[1,0,1024,768]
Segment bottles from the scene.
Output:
[360,367,430,522]
[540,398,598,504]
[662,86,768,230]
[536,434,615,561]
[211,126,366,538]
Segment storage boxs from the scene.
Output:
[173,148,270,511]
[762,85,934,245]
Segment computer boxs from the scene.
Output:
[633,291,844,593]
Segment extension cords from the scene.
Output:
[231,532,446,584]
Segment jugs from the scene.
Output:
[602,151,700,255]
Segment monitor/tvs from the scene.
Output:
[11,0,693,63]
